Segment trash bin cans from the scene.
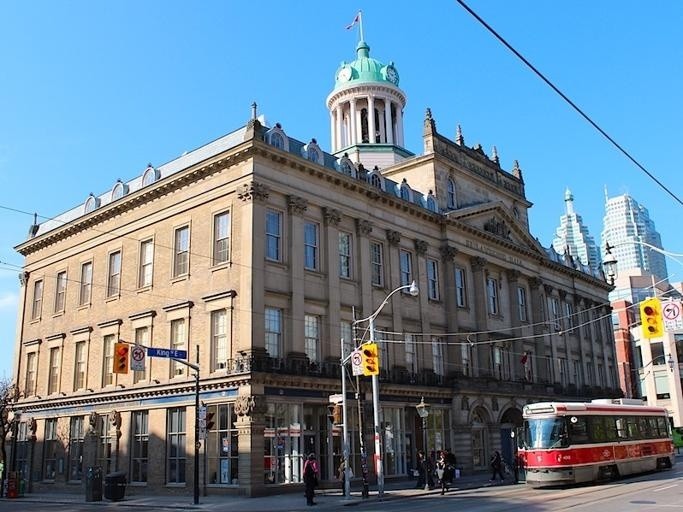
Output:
[85,466,128,501]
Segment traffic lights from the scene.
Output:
[205,411,214,431]
[639,298,664,339]
[325,404,339,425]
[361,343,379,377]
[113,342,128,374]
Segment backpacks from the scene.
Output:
[304,464,316,484]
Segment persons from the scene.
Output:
[415,447,457,496]
[301,452,320,506]
[512,451,522,483]
[337,456,352,495]
[487,450,506,483]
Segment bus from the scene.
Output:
[510,397,675,490]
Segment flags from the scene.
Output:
[345,15,359,31]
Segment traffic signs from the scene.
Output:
[146,348,186,359]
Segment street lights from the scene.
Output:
[10,411,21,479]
[414,395,430,490]
[628,354,673,401]
[350,280,419,497]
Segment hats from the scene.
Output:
[309,453,316,458]
